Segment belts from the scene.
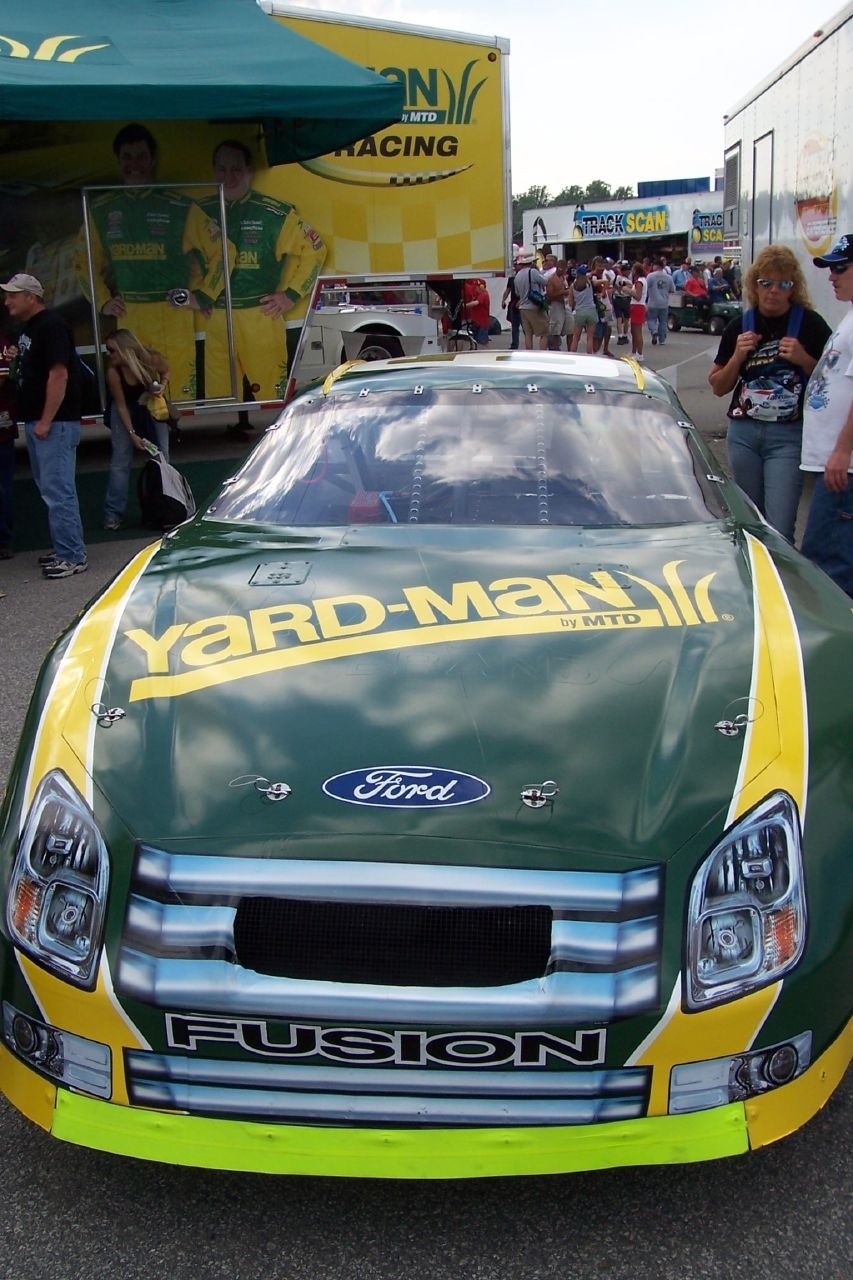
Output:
[214,296,267,310]
[123,292,175,303]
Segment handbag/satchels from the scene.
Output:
[161,377,180,428]
[527,269,545,307]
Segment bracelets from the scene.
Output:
[128,430,135,435]
[162,383,166,390]
[631,291,633,295]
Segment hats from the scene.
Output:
[577,267,587,273]
[520,253,539,263]
[0,274,45,297]
[812,234,853,268]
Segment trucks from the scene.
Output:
[0,0,514,428]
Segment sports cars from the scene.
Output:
[0,349,853,1184]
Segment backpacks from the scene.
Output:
[137,451,196,530]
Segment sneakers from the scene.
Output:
[39,552,88,565]
[104,518,124,530]
[42,556,88,577]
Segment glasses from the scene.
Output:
[105,345,119,354]
[756,278,795,289]
[829,263,853,273]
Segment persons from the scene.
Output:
[501,240,743,362]
[69,123,237,408]
[189,140,327,406]
[708,246,833,545]
[104,329,169,529]
[0,274,88,578]
[446,280,490,352]
[799,234,853,598]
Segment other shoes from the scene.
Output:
[652,330,658,344]
[603,352,614,357]
[0,548,14,559]
[617,336,629,344]
[660,342,666,344]
[631,353,645,362]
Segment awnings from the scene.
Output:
[0,0,406,168]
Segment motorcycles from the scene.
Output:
[668,283,742,336]
